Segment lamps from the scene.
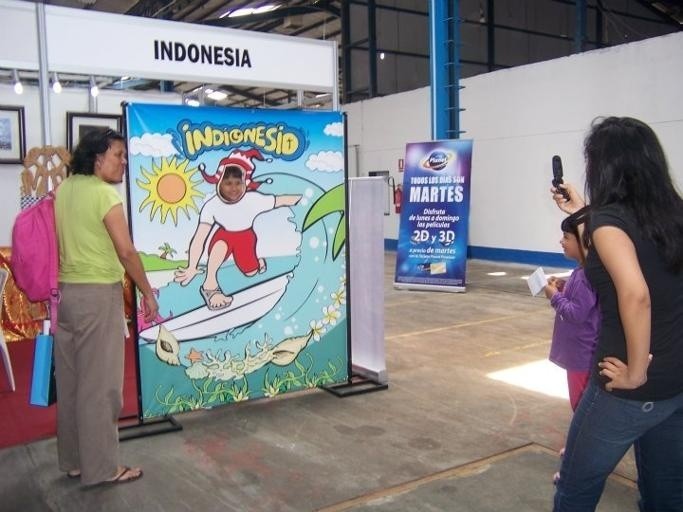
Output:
[10,69,100,97]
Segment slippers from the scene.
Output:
[66,464,143,484]
[200,283,231,311]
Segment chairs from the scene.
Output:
[0,268,16,392]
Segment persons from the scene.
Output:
[545,214,604,485]
[551,115,683,512]
[49,126,159,490]
[219,166,244,202]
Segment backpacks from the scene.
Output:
[11,193,59,304]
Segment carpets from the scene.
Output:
[0,323,140,450]
[486,359,570,403]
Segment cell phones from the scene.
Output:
[551,155,571,204]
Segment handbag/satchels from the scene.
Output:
[28,332,58,408]
